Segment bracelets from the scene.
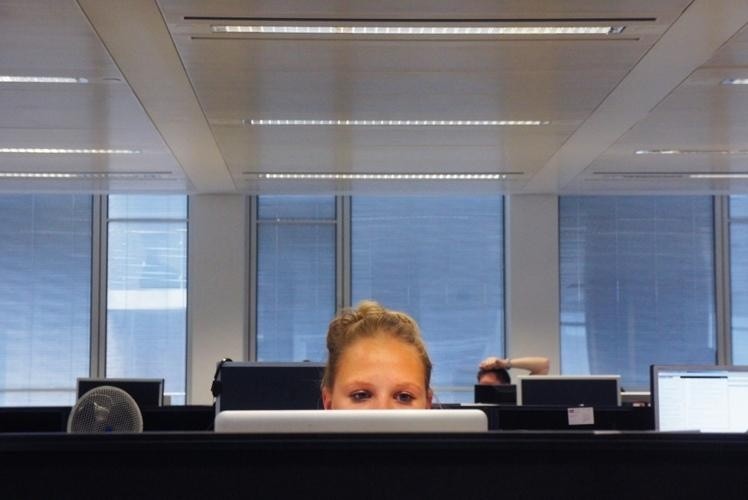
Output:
[505,358,514,371]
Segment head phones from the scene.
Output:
[210,358,233,397]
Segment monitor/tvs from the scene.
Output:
[517,375,621,407]
[650,365,748,432]
[215,360,330,411]
[474,384,516,404]
[74,378,164,408]
[214,409,488,434]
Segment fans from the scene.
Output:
[68,386,144,434]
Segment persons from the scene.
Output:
[322,301,432,411]
[475,355,550,386]
